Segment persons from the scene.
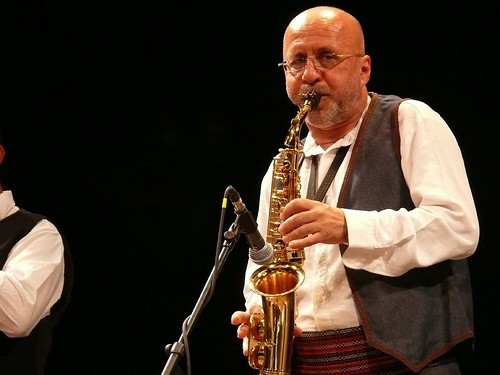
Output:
[1,142,66,375]
[231,6,480,375]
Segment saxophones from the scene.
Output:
[245,92,321,374]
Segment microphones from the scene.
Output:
[228,186,273,264]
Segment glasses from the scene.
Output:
[277,53,363,73]
